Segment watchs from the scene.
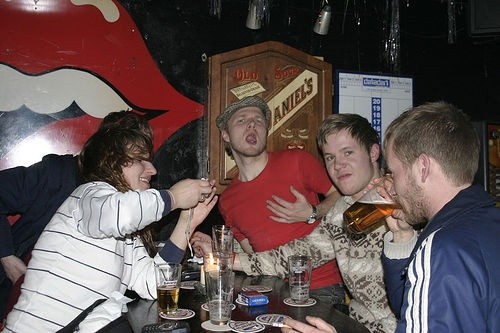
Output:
[305,206,316,225]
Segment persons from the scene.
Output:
[0,110,218,333]
[190,113,425,333]
[280,103,500,333]
[217,96,346,316]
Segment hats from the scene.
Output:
[215,95,271,128]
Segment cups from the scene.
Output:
[343,177,402,234]
[203,252,234,308]
[206,270,235,326]
[288,255,312,304]
[154,262,181,313]
[212,225,234,252]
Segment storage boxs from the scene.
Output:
[238,290,268,307]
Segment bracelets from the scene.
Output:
[165,190,175,211]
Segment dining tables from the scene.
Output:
[126,267,371,333]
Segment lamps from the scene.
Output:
[246,0,263,30]
[314,0,332,36]
